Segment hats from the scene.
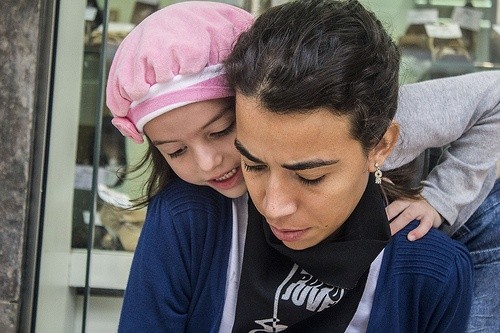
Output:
[105,0,254,143]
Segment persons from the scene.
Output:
[118,0,475,332]
[106,0,499,332]
